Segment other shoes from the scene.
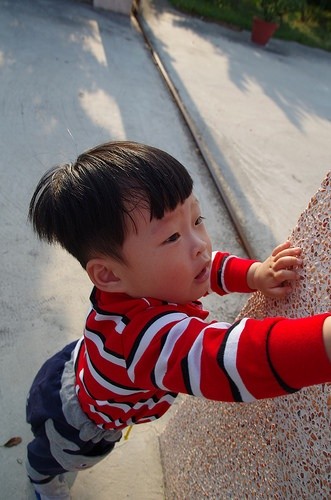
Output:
[27,470,71,500]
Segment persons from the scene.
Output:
[23,140,331,500]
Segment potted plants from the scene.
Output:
[251,0,302,46]
